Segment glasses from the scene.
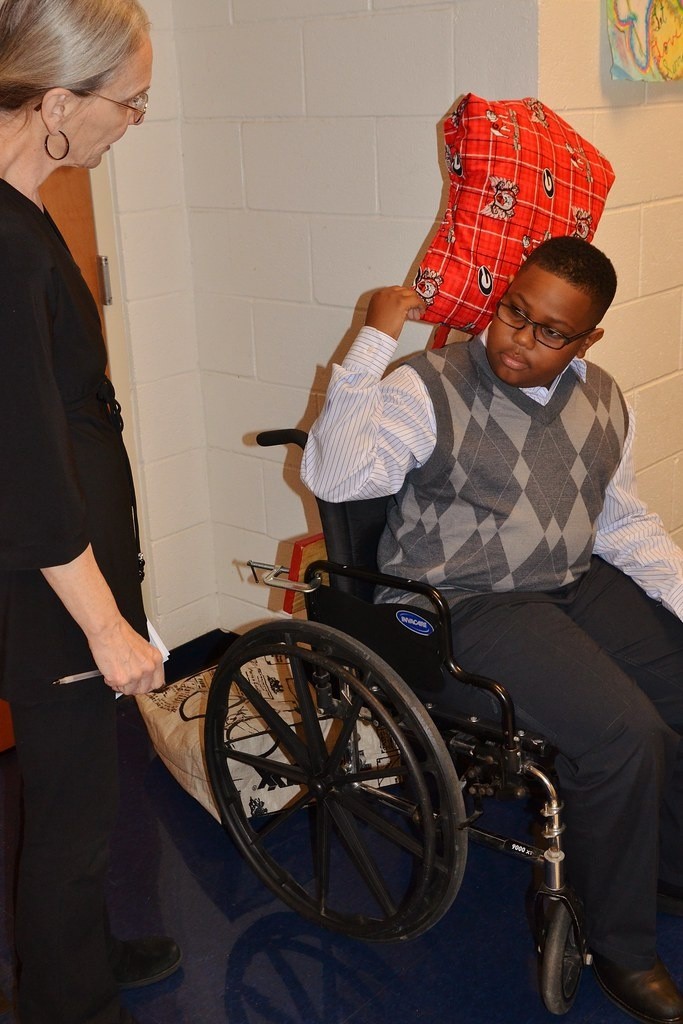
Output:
[496,287,597,351]
[34,88,149,124]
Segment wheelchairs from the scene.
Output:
[202,429,606,1015]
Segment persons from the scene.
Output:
[0,0,190,998]
[296,235,683,1020]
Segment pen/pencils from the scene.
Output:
[52,670,104,684]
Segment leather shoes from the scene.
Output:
[588,940,683,1024]
[105,937,183,989]
[657,878,683,900]
